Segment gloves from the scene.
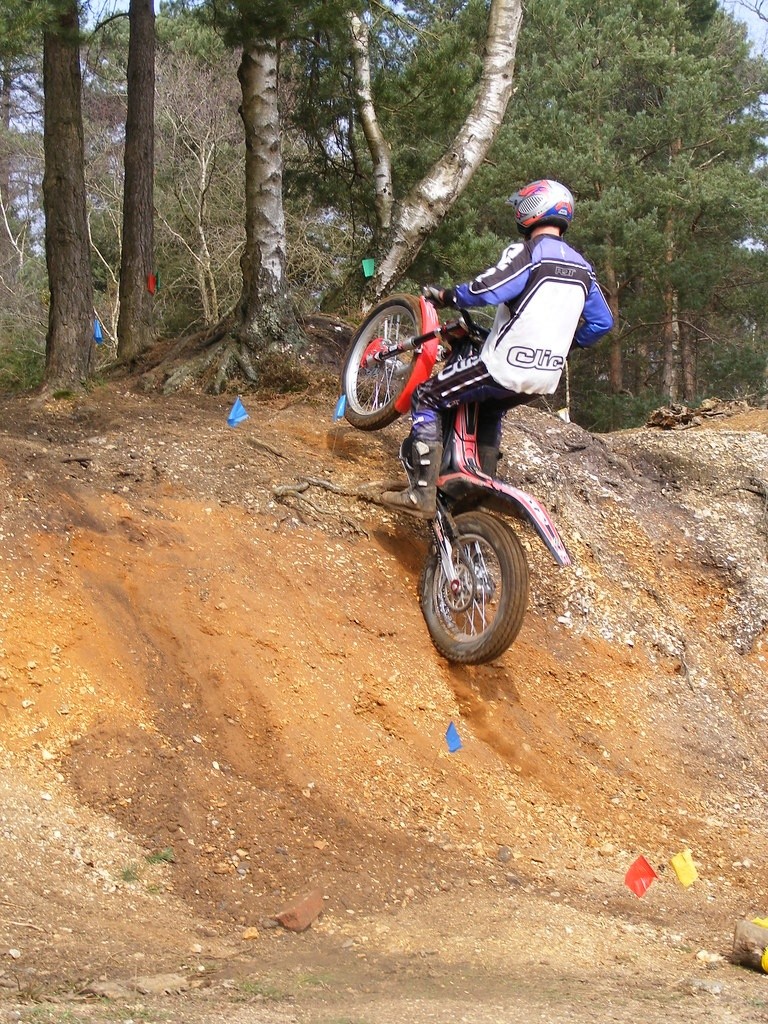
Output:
[424,284,447,310]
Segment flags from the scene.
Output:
[228,397,250,427]
[147,272,161,294]
[446,722,462,752]
[362,259,375,277]
[334,395,345,420]
[625,856,659,898]
[671,852,698,887]
[93,320,103,344]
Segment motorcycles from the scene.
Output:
[340,286,572,666]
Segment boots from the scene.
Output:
[379,441,443,520]
[478,442,500,480]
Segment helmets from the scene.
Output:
[513,179,574,235]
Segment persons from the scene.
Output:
[380,179,615,520]
